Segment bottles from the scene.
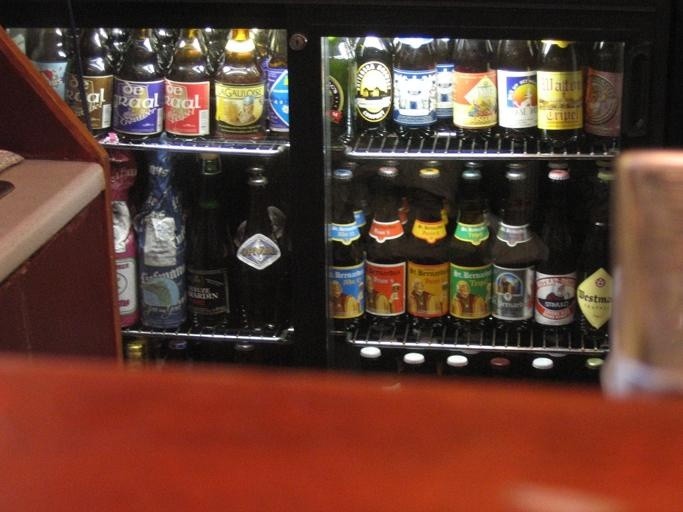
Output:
[365,160,412,333]
[4,22,291,139]
[435,38,454,126]
[406,160,450,337]
[452,38,498,145]
[328,160,371,332]
[533,160,580,338]
[492,160,535,337]
[111,152,297,363]
[497,39,537,144]
[393,37,438,141]
[448,159,498,337]
[583,41,624,150]
[329,37,354,147]
[577,159,616,344]
[536,40,583,149]
[351,36,393,140]
